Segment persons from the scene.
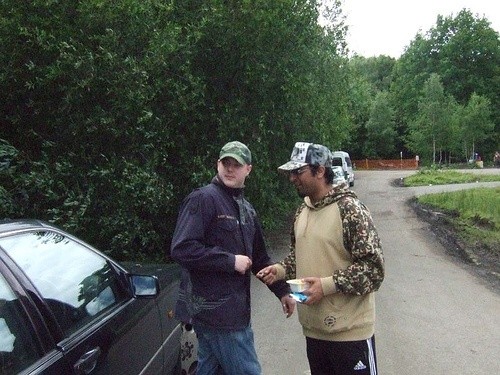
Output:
[472,151,500,168]
[171,141,295,375]
[255,141,385,375]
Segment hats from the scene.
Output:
[278,142,331,171]
[219,141,252,166]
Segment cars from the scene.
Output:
[329,150,355,189]
[0,218,198,375]
